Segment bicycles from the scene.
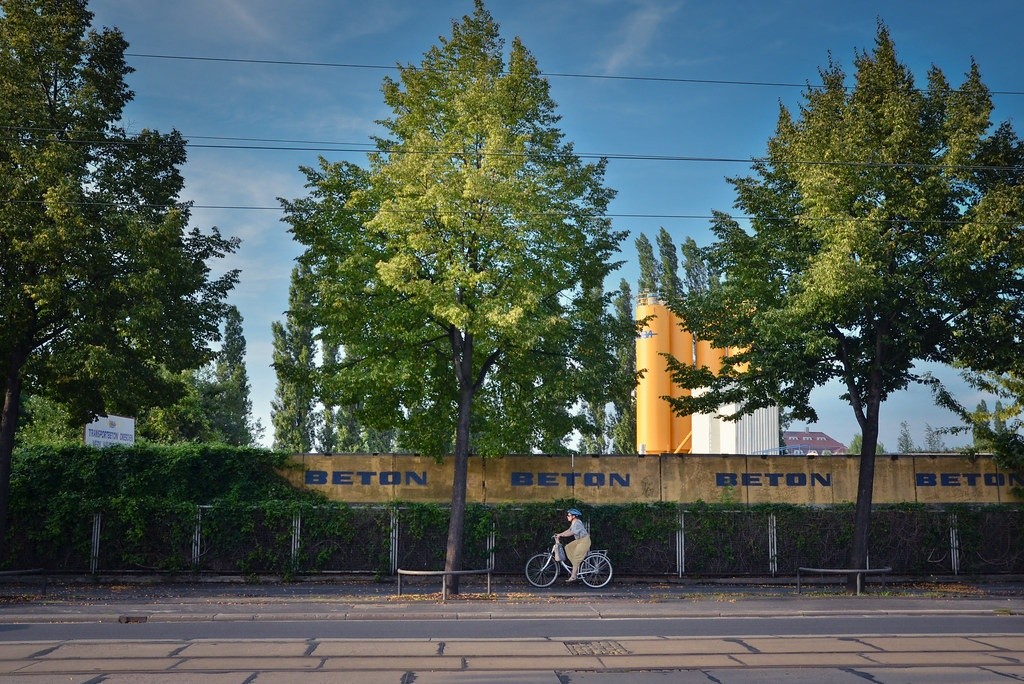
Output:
[525,533,617,589]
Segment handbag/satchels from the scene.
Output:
[555,537,566,561]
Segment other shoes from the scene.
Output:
[565,577,576,582]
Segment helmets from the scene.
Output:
[567,509,582,515]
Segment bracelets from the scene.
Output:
[562,535,563,537]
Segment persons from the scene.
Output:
[554,509,591,583]
